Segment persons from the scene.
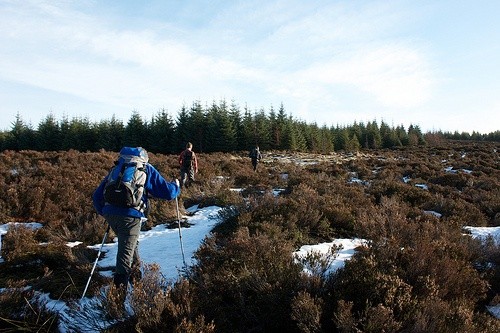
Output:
[90,147,181,309]
[178,143,198,184]
[252,146,262,173]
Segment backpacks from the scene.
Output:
[101,146,152,209]
[182,149,194,168]
[250,148,260,162]
